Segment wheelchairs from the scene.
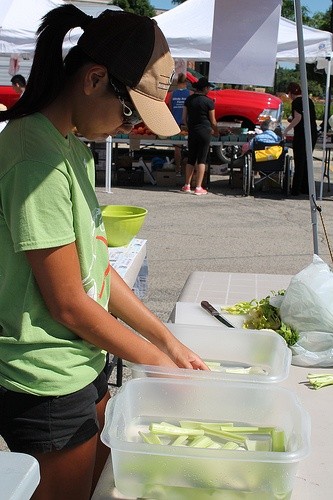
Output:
[241,140,292,197]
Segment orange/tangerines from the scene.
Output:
[131,125,189,136]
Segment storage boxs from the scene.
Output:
[116,127,251,142]
[123,321,292,384]
[100,377,310,500]
[117,166,144,186]
[232,170,244,187]
[156,171,176,188]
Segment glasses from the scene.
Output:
[111,82,142,128]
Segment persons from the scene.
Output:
[182,78,218,195]
[285,83,318,199]
[10,74,27,100]
[168,73,194,176]
[0,3,212,500]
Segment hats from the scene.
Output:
[286,82,301,95]
[198,77,214,88]
[79,9,181,138]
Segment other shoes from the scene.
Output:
[194,187,206,195]
[181,186,191,193]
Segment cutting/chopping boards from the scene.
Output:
[175,301,250,330]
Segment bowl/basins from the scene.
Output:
[99,205,148,247]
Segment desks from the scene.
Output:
[72,134,333,194]
[103,237,149,385]
[91,270,333,500]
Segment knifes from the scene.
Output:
[200,300,236,328]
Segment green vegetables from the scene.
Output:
[306,373,333,390]
[220,289,298,345]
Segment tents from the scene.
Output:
[0,0,84,86]
[151,0,333,200]
